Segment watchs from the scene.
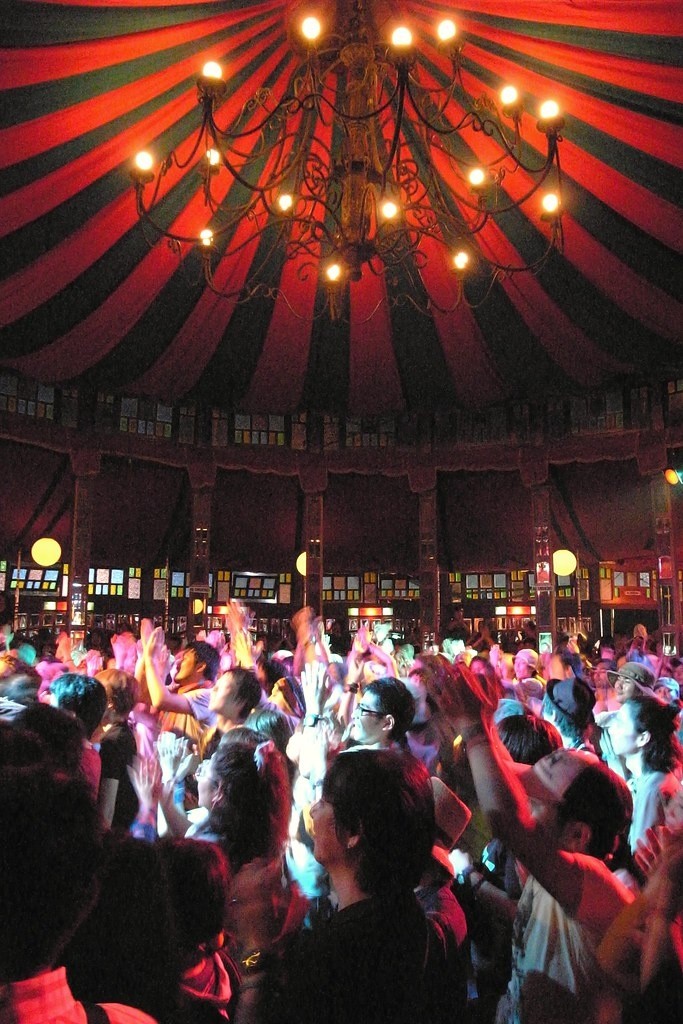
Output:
[456,864,473,887]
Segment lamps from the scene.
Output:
[131,0,566,323]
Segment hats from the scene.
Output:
[547,676,597,724]
[503,747,634,835]
[653,677,679,697]
[276,676,306,720]
[512,649,539,676]
[606,662,658,698]
[272,650,294,663]
[427,776,471,878]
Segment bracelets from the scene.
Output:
[240,977,265,992]
[239,947,265,972]
[462,720,488,746]
[466,874,486,893]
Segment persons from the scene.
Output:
[0,601,683,1024]
[226,751,469,1024]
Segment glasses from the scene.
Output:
[195,764,218,786]
[355,703,386,716]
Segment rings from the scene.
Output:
[230,896,238,906]
